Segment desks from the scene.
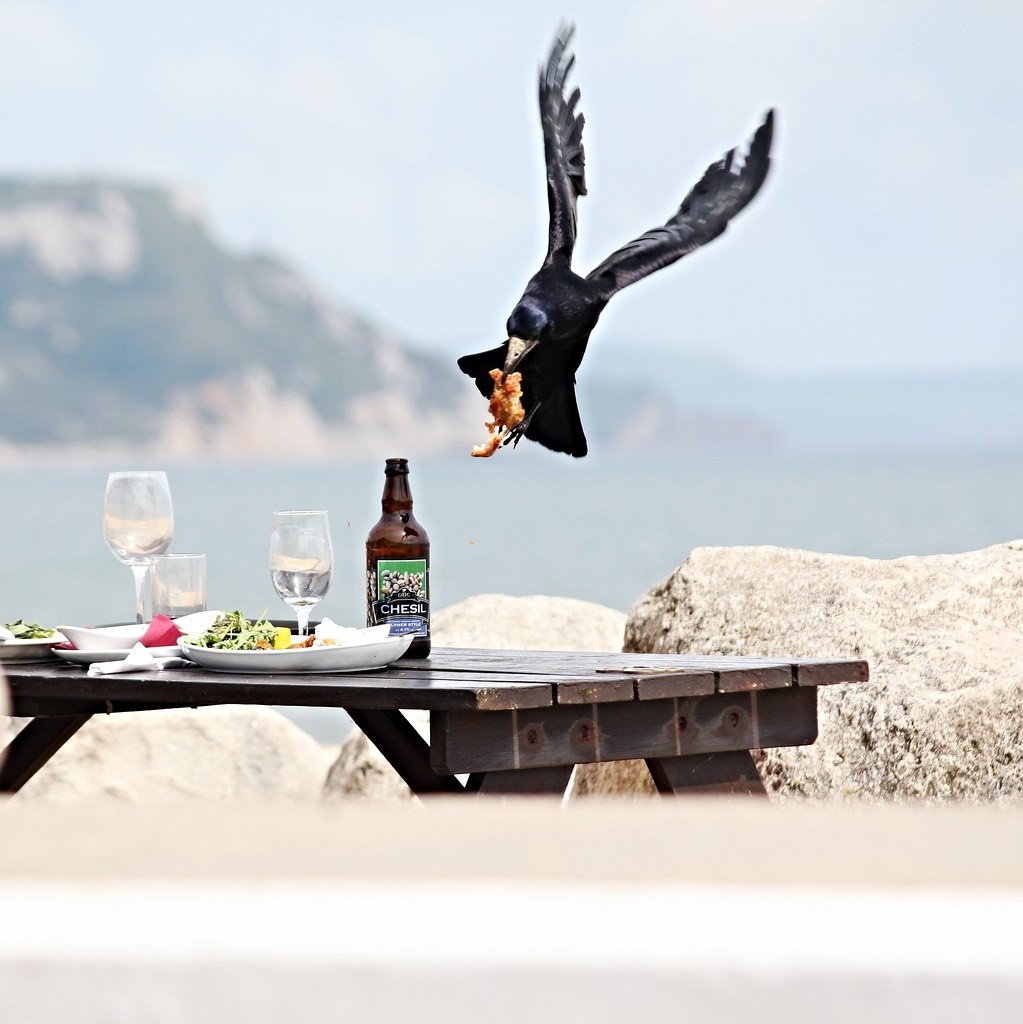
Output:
[1,648,869,797]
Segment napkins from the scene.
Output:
[86,642,197,676]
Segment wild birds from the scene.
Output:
[458,20,775,457]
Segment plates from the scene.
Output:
[0,631,70,660]
[177,633,414,674]
[51,644,183,664]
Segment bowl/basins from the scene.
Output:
[58,624,149,651]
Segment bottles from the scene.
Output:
[365,458,431,659]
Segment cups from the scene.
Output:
[151,554,206,620]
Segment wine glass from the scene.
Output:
[271,509,334,636]
[101,469,174,624]
